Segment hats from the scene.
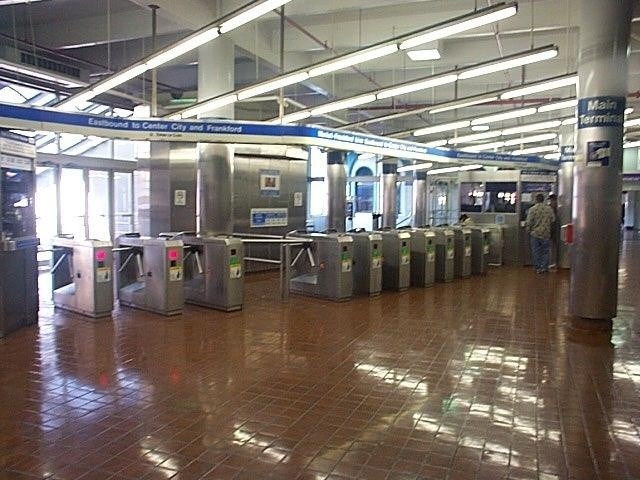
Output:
[546,190,558,200]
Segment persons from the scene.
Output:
[525,193,560,275]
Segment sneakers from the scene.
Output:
[536,264,557,274]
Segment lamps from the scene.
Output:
[423,133,560,157]
[263,43,555,124]
[426,77,577,115]
[412,96,576,138]
[164,2,520,121]
[48,2,288,108]
